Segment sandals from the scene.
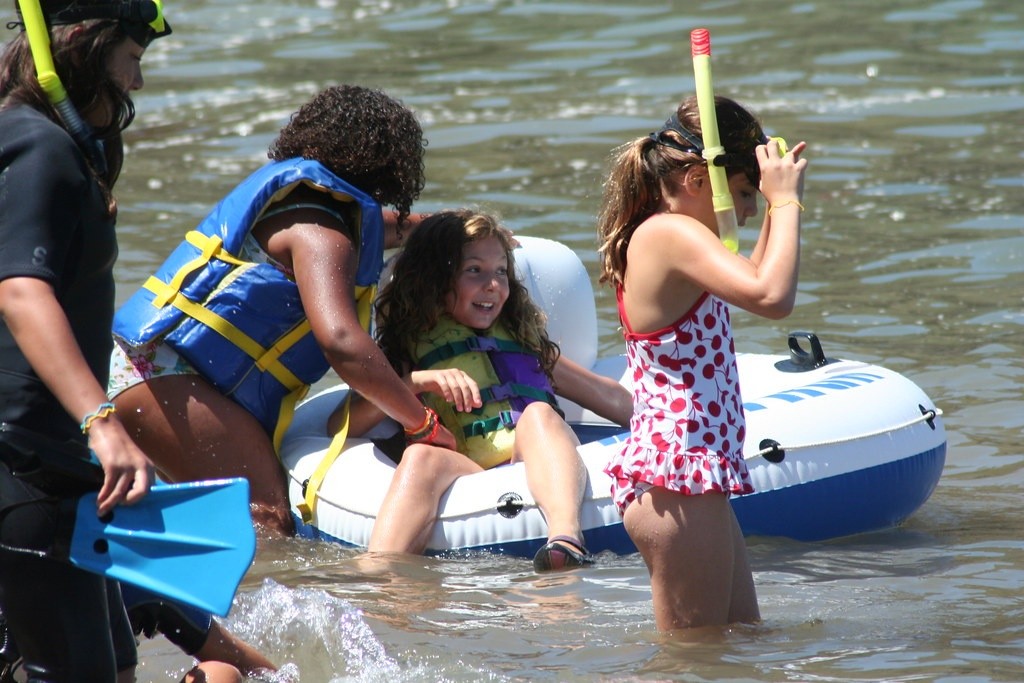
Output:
[533,536,595,574]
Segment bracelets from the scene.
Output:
[769,200,804,215]
[80,403,116,434]
[404,406,438,444]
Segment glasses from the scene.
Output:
[742,150,762,192]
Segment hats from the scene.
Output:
[17,0,172,48]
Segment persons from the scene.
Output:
[106,86,456,528]
[328,211,633,570]
[599,96,807,629]
[0,1,278,683]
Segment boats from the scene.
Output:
[276,349,947,559]
